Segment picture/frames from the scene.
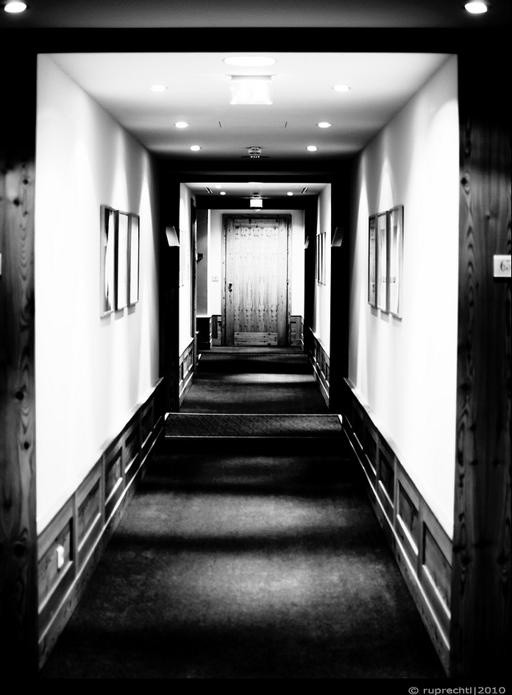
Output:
[100,205,140,319]
[316,232,326,285]
[368,205,403,319]
